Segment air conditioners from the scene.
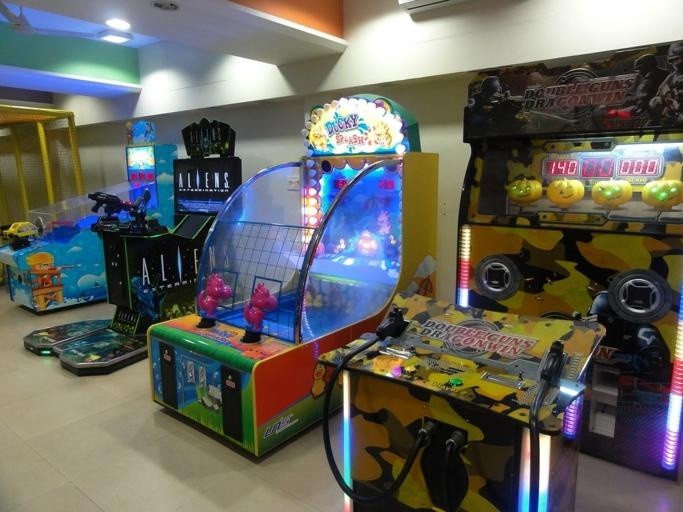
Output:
[397,0,473,14]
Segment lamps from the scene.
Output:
[97,29,133,45]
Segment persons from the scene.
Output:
[647,42,683,127]
[628,53,671,124]
[465,75,525,134]
